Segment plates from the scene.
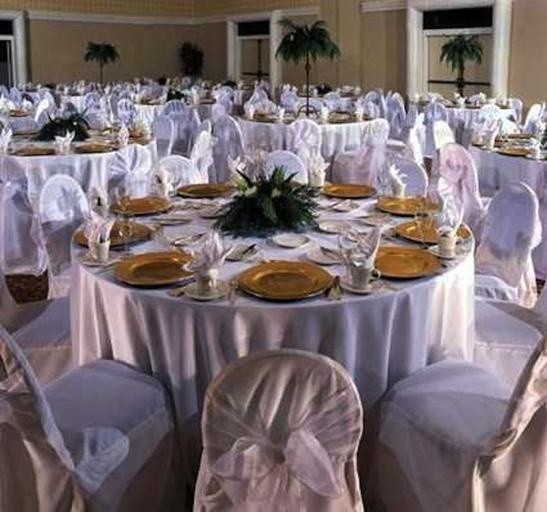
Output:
[472,133,546,161]
[12,127,149,156]
[76,221,153,247]
[239,259,334,301]
[108,195,172,216]
[254,110,373,124]
[320,182,376,200]
[396,218,469,246]
[371,244,440,284]
[10,112,29,117]
[376,193,442,218]
[72,181,470,302]
[175,182,236,198]
[115,253,195,288]
